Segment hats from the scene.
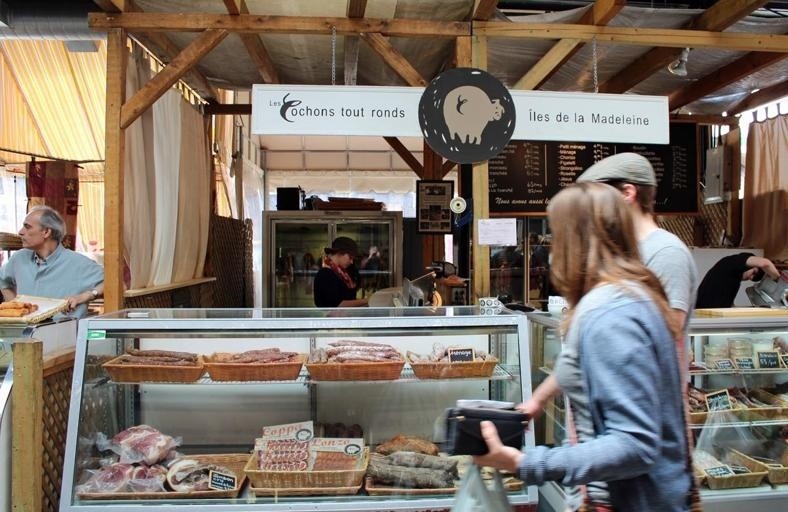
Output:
[576,152,657,187]
[332,238,361,257]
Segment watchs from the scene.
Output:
[88,288,100,298]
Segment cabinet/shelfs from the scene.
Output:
[260,210,403,309]
[489,210,553,312]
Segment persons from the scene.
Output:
[1,206,107,322]
[472,178,696,510]
[310,236,377,310]
[696,252,781,308]
[488,229,560,313]
[511,151,691,510]
[360,244,385,292]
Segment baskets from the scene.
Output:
[407,350,498,378]
[688,384,788,490]
[306,355,406,380]
[102,355,204,382]
[78,453,249,499]
[243,446,370,494]
[205,351,304,381]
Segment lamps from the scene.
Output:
[668,47,689,77]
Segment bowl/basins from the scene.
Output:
[547,303,568,318]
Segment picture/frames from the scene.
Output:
[415,180,454,235]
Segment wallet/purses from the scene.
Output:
[432,408,531,455]
